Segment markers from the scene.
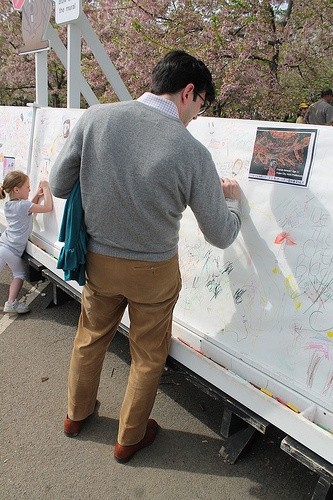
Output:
[28,238,58,262]
[177,335,228,372]
[248,379,302,415]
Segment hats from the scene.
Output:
[299,103,309,110]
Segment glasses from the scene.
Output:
[192,90,210,116]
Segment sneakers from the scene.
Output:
[3,298,30,313]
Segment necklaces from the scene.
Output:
[9,197,19,201]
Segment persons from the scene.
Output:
[49,49,242,465]
[304,88,333,126]
[0,171,53,313]
[296,102,309,124]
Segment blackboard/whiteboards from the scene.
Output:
[1,102,333,463]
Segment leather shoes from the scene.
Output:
[63,399,98,438]
[114,419,159,463]
